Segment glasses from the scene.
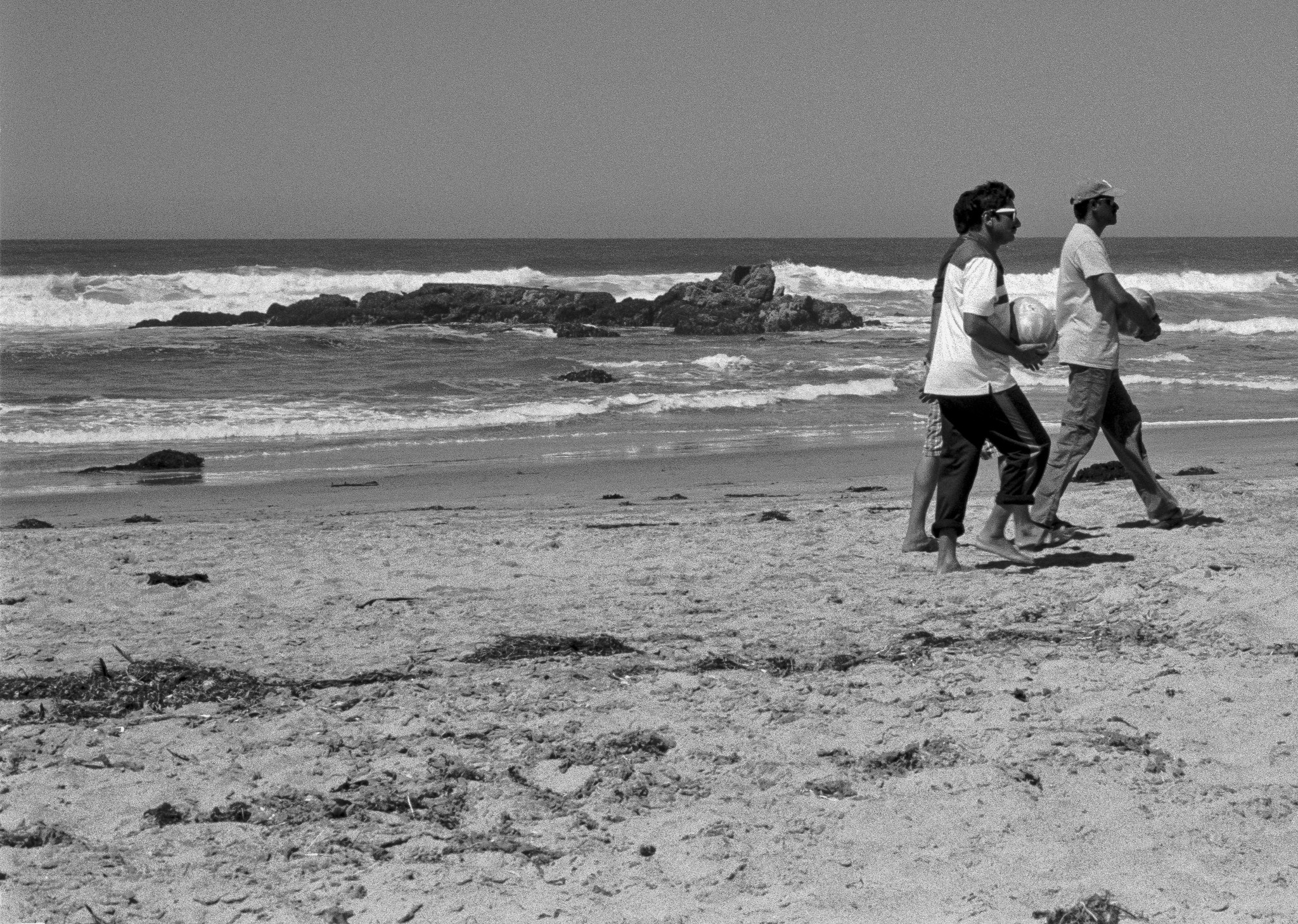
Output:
[992,208,1017,221]
[1100,197,1116,205]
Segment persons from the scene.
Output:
[1029,178,1204,533]
[899,178,1072,576]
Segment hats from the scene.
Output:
[1071,181,1124,203]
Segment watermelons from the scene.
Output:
[1115,288,1155,338]
[1008,296,1057,354]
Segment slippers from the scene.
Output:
[1030,528,1073,551]
[1051,520,1079,533]
[1155,508,1204,528]
